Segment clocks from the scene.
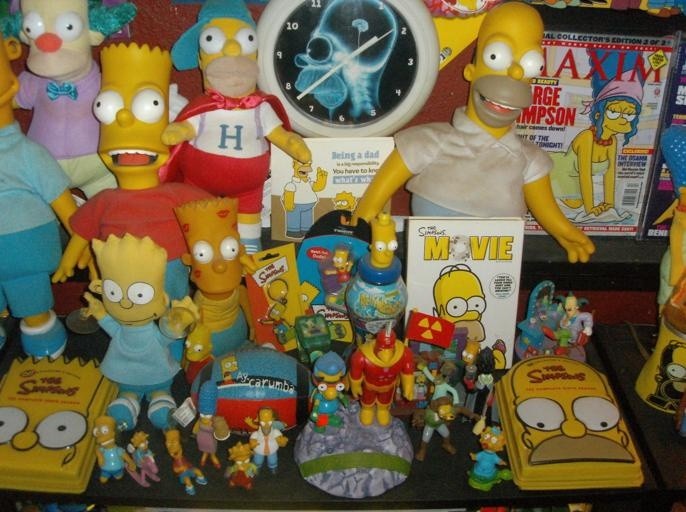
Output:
[255,0,437,138]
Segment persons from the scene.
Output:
[1,1,685,500]
[0,1,685,498]
